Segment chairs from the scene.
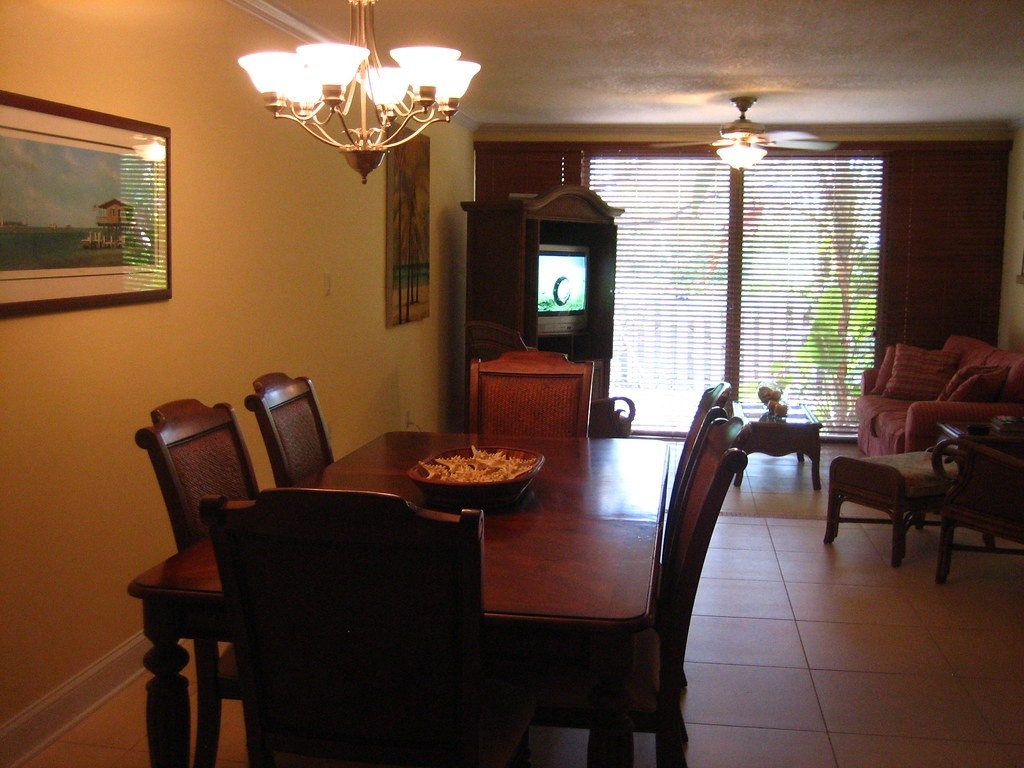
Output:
[133,400,259,768]
[465,319,635,438]
[244,372,336,487]
[931,438,1024,585]
[496,381,747,768]
[198,486,535,767]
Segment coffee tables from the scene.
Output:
[731,400,823,491]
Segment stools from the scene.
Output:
[822,445,996,568]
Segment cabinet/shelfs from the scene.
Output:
[460,182,625,435]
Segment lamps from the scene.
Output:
[716,141,767,169]
[236,0,481,186]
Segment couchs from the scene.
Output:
[856,334,1024,456]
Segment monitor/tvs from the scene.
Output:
[537,244,590,332]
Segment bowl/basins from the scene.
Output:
[408,445,545,508]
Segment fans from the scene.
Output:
[649,96,839,152]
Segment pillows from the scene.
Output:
[937,364,999,402]
[870,345,895,395]
[947,364,1011,402]
[882,343,954,402]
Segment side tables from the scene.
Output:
[934,420,1024,457]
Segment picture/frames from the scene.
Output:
[0,90,173,320]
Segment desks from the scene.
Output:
[124,432,670,767]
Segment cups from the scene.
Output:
[768,401,788,417]
